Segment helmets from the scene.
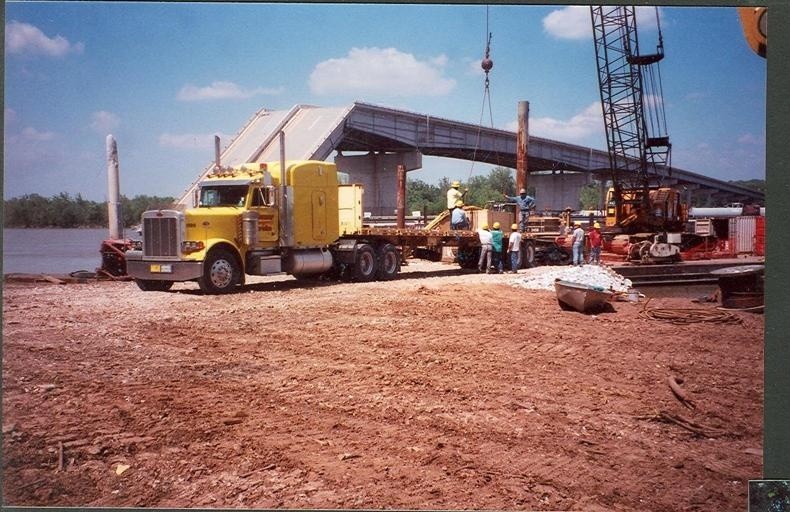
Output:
[481,223,489,230]
[451,181,461,188]
[454,200,464,207]
[520,188,527,194]
[574,221,581,226]
[594,223,601,228]
[511,223,518,229]
[493,222,501,229]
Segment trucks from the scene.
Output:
[122,126,594,296]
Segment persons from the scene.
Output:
[587,222,603,266]
[502,188,537,233]
[569,220,586,268]
[475,223,495,275]
[506,224,523,275]
[446,181,468,216]
[451,200,472,230]
[490,222,507,275]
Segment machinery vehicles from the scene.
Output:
[591,4,691,234]
